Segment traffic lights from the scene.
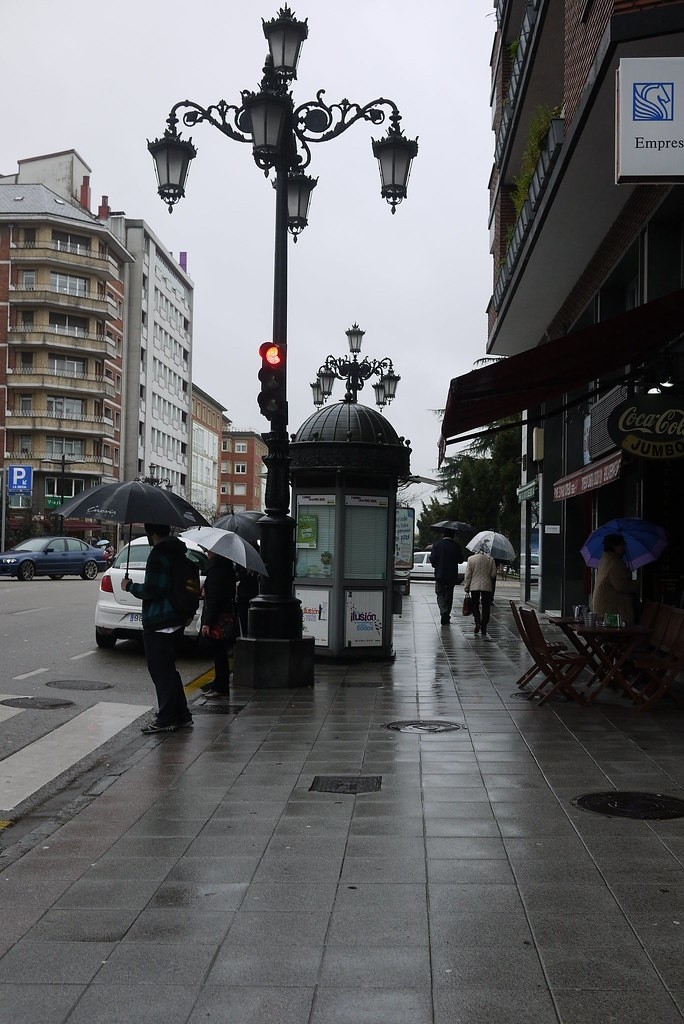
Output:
[258,341,286,421]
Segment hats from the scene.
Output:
[601,533,626,545]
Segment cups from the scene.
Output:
[574,605,587,620]
[583,612,598,627]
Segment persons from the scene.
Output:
[592,534,642,629]
[101,543,114,556]
[488,548,504,605]
[464,546,498,634]
[198,550,242,699]
[122,523,200,734]
[429,528,464,626]
[235,564,260,636]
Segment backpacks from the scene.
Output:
[163,547,200,617]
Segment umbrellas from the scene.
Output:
[189,526,270,577]
[216,509,266,543]
[429,519,476,537]
[466,531,516,561]
[96,540,110,546]
[48,477,213,579]
[579,517,668,582]
[84,536,98,542]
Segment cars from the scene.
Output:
[410,551,468,585]
[94,536,241,649]
[501,553,540,583]
[0,536,108,581]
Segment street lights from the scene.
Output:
[144,5,419,692]
[41,454,86,536]
[309,323,402,412]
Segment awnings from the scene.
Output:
[438,289,684,470]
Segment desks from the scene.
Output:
[548,616,605,681]
[577,625,655,707]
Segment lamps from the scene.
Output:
[647,353,684,395]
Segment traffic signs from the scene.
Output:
[8,465,33,490]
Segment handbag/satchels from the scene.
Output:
[197,606,241,641]
[462,597,473,616]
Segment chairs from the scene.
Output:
[601,602,684,711]
[509,600,597,707]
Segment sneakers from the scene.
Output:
[178,717,194,728]
[141,720,175,734]
[201,688,230,697]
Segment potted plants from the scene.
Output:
[492,103,564,312]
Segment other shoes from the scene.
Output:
[474,623,481,633]
[481,627,486,633]
[490,602,494,605]
[441,611,450,625]
